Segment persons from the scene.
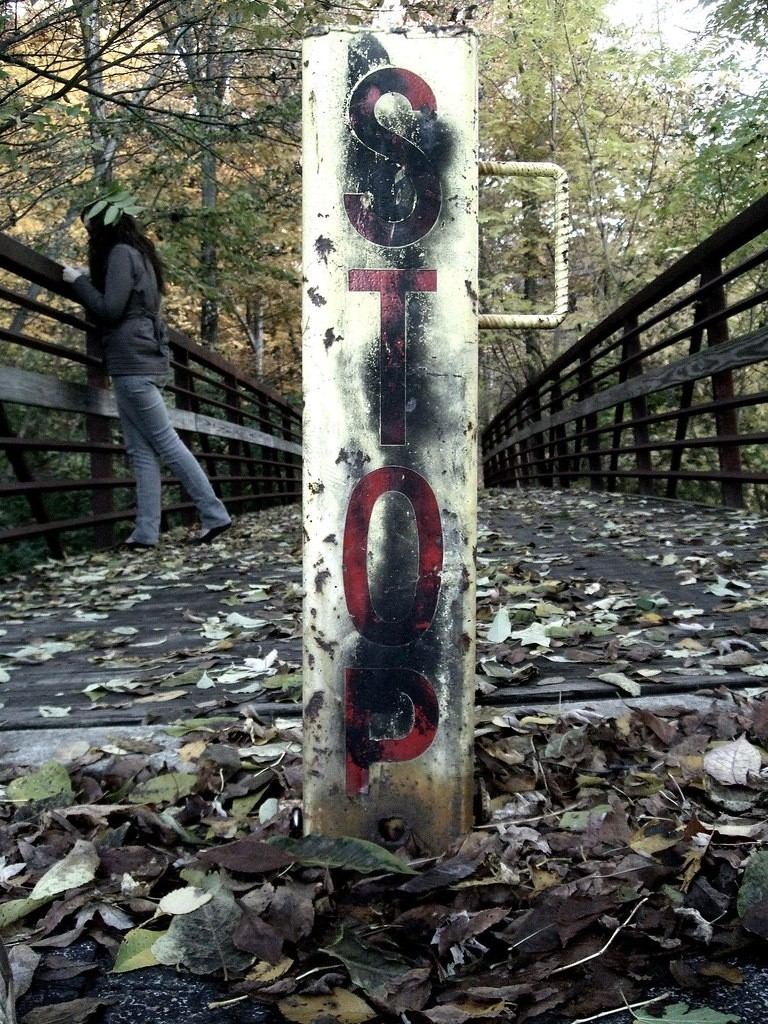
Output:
[56,200,234,556]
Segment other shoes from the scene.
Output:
[188,522,232,546]
[111,541,155,551]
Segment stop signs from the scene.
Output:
[306,32,575,857]
